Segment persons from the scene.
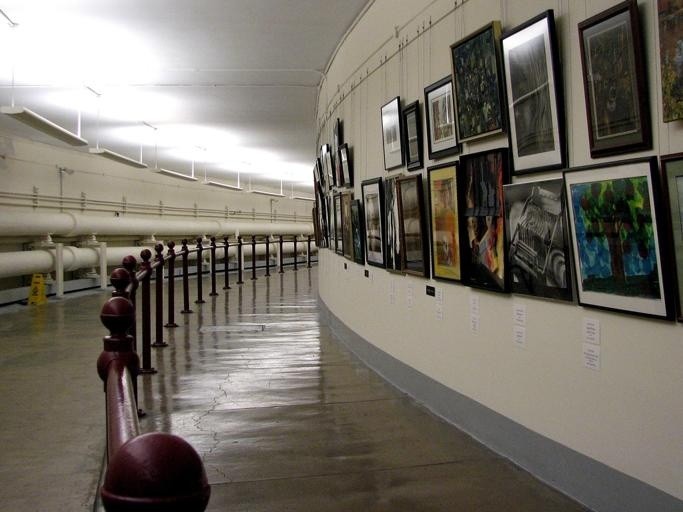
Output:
[439,180,452,217]
[441,235,451,265]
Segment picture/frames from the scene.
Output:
[396,174,429,279]
[311,143,334,252]
[340,190,355,261]
[384,173,406,276]
[450,19,507,144]
[426,158,464,287]
[349,199,366,266]
[502,177,579,306]
[576,0,655,160]
[458,147,511,297]
[332,118,342,188]
[380,96,405,170]
[655,0,682,125]
[400,100,425,172]
[661,150,682,325]
[423,74,462,160]
[561,156,678,325]
[358,176,386,269]
[498,8,569,177]
[337,142,352,188]
[334,192,345,257]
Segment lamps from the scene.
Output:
[246,174,286,198]
[0,66,89,148]
[87,115,149,170]
[201,164,244,192]
[154,143,199,183]
[289,184,316,202]
